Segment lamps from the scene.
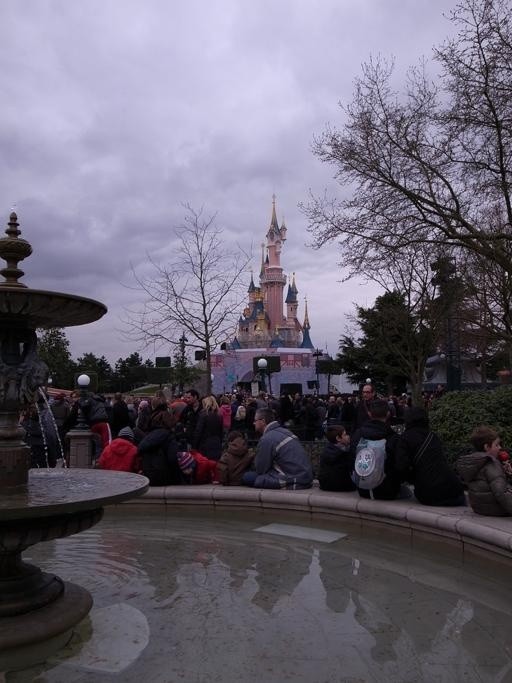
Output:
[258,358,268,397]
[76,373,91,430]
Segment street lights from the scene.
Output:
[427,254,461,393]
[257,357,269,397]
[312,348,324,395]
[178,327,189,392]
[366,376,372,386]
[74,371,93,430]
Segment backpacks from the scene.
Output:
[350,436,386,491]
[233,404,247,421]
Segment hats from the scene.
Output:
[175,451,195,471]
[138,400,149,409]
[117,425,135,442]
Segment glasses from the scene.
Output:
[362,391,373,395]
[253,419,263,422]
